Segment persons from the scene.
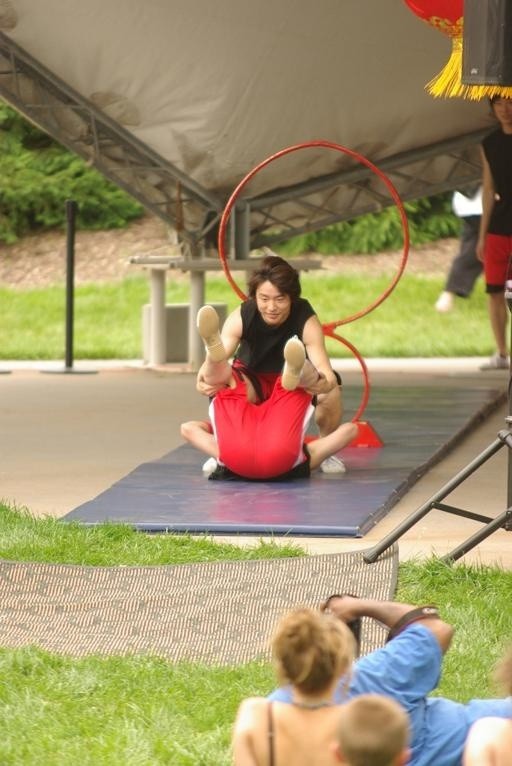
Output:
[233,587,512,766]
[180,302,358,478]
[198,254,349,479]
[476,93,511,372]
[429,184,484,313]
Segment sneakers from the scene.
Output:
[319,453,346,474]
[201,456,231,481]
[480,353,511,371]
[281,338,306,392]
[437,291,457,313]
[196,306,227,364]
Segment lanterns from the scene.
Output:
[409,0,510,98]
